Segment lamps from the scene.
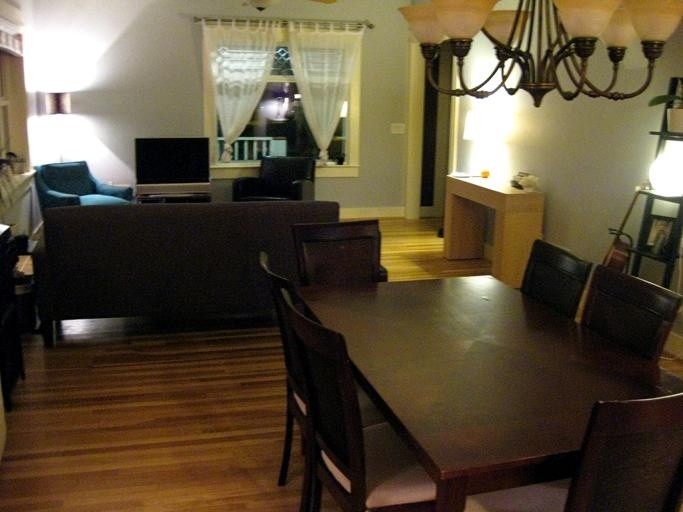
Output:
[458,109,494,178]
[395,0,682,112]
[39,88,77,120]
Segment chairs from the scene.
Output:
[255,249,382,512]
[288,216,387,285]
[275,287,438,512]
[578,263,681,386]
[466,390,683,512]
[263,139,287,157]
[518,235,597,321]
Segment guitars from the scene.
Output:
[602,185,651,271]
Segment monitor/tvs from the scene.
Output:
[134,137,211,195]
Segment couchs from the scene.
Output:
[35,160,138,209]
[27,194,342,349]
[230,152,318,201]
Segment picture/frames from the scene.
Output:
[636,213,676,256]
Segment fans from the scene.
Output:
[243,0,342,14]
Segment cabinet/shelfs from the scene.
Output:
[0,224,27,410]
[624,72,681,289]
[0,13,37,236]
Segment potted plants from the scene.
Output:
[645,84,682,134]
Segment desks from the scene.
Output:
[439,170,548,291]
[291,268,681,511]
